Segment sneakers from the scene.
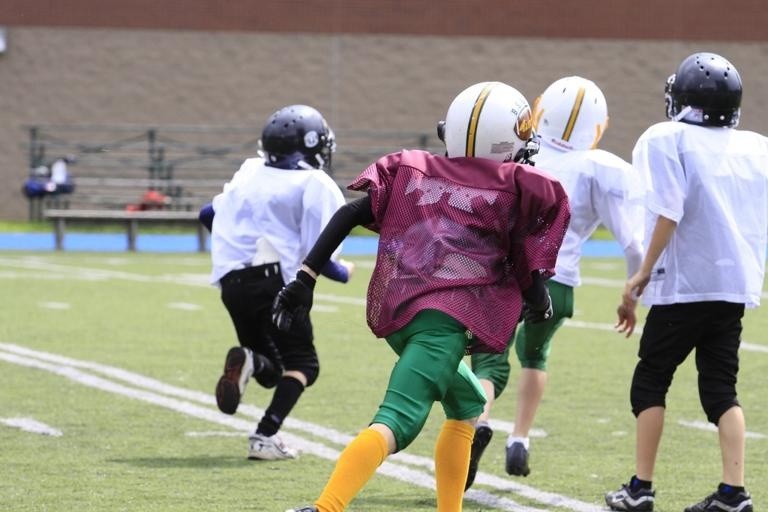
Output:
[505,434,531,477]
[214,346,255,414]
[464,419,494,492]
[683,490,753,512]
[247,432,296,460]
[605,483,656,512]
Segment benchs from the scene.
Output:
[21,116,447,227]
[44,205,209,255]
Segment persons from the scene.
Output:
[604,53,768,512]
[464,75,645,493]
[198,105,353,461]
[270,80,570,511]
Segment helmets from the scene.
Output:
[260,104,338,172]
[436,80,540,166]
[662,52,744,129]
[532,74,610,151]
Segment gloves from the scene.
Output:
[521,268,553,325]
[270,270,317,338]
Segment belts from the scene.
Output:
[220,262,281,288]
